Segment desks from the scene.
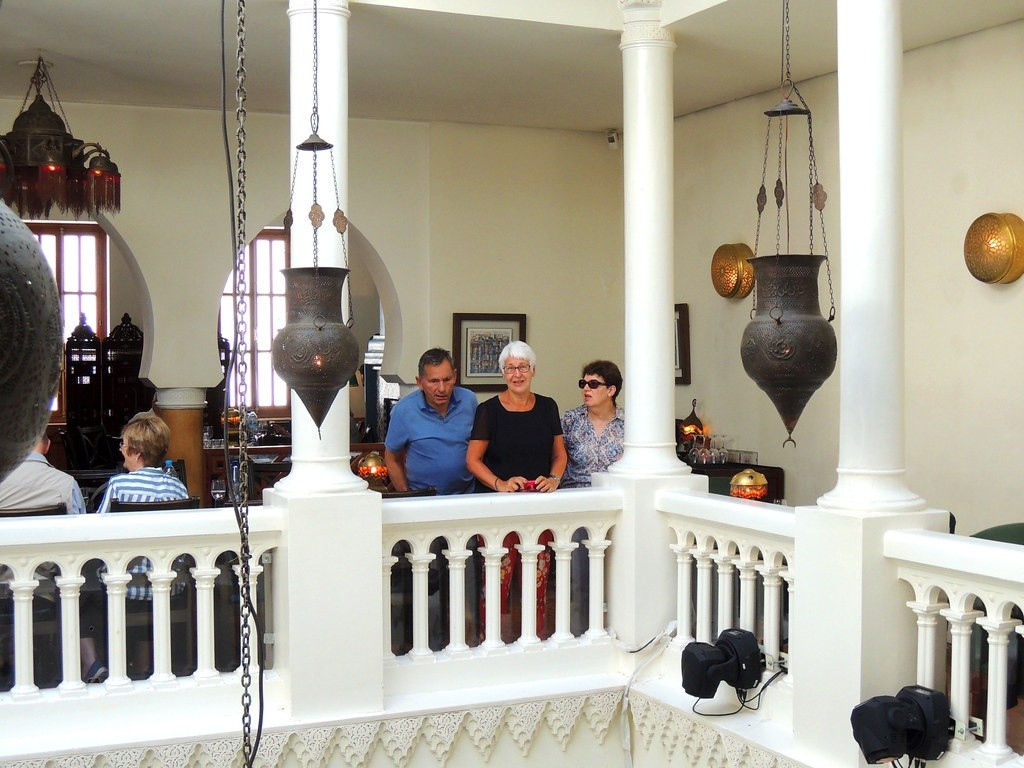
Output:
[686,462,785,504]
[203,443,406,511]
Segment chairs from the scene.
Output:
[0,458,202,684]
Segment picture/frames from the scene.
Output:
[674,303,693,385]
[453,314,528,395]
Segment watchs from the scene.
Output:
[548,474,561,485]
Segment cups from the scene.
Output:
[210,479,226,500]
[202,426,227,449]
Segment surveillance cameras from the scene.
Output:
[606,131,619,144]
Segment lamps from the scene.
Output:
[681,398,704,457]
[1,59,121,225]
[712,244,753,299]
[851,685,956,765]
[272,0,358,440]
[681,631,763,699]
[742,0,838,448]
[962,213,1023,284]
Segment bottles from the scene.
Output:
[228,466,242,502]
[247,408,259,446]
[163,460,177,477]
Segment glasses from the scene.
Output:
[119,442,129,449]
[503,366,531,374]
[578,379,607,389]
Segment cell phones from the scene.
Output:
[519,481,541,491]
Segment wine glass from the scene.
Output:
[688,434,729,464]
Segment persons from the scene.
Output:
[77,414,190,685]
[560,359,625,635]
[385,348,479,654]
[0,432,87,619]
[465,339,567,647]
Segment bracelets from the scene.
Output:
[495,478,500,491]
